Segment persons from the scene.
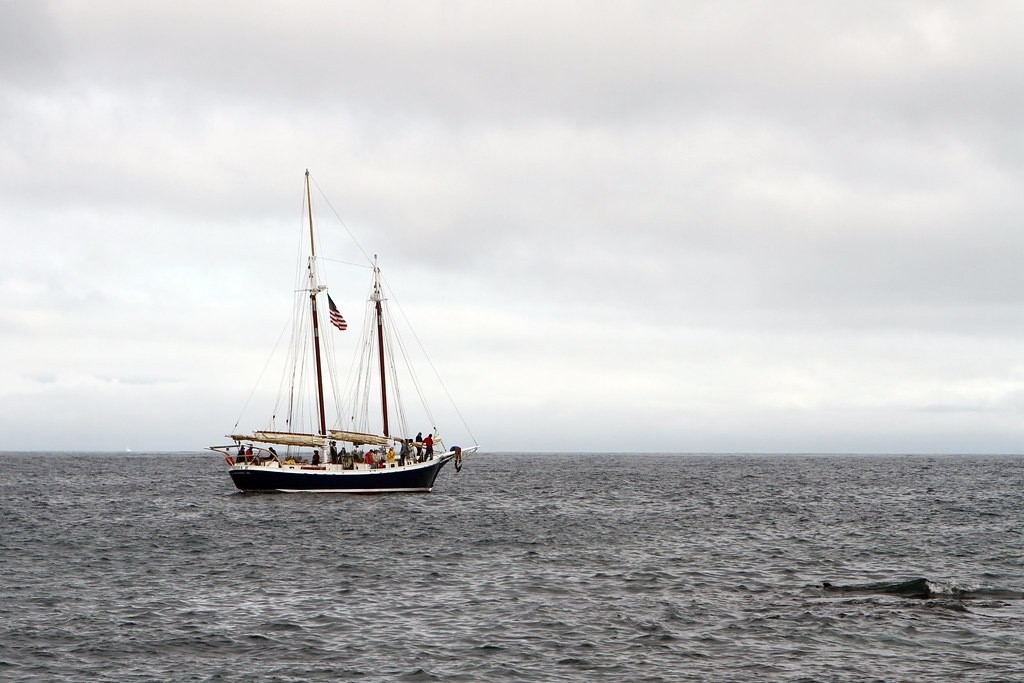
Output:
[269,447,277,459]
[451,446,461,460]
[329,439,394,469]
[400,440,406,466]
[237,447,245,463]
[246,444,258,464]
[312,450,319,464]
[423,434,433,461]
[416,432,423,456]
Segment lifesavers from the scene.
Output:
[226,456,235,466]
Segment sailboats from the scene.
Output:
[202,167,482,495]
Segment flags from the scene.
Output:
[328,295,347,330]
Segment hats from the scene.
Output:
[268,447,273,449]
[390,446,394,450]
[342,447,345,449]
[241,446,245,449]
[370,450,374,452]
[314,450,319,454]
[331,441,336,443]
